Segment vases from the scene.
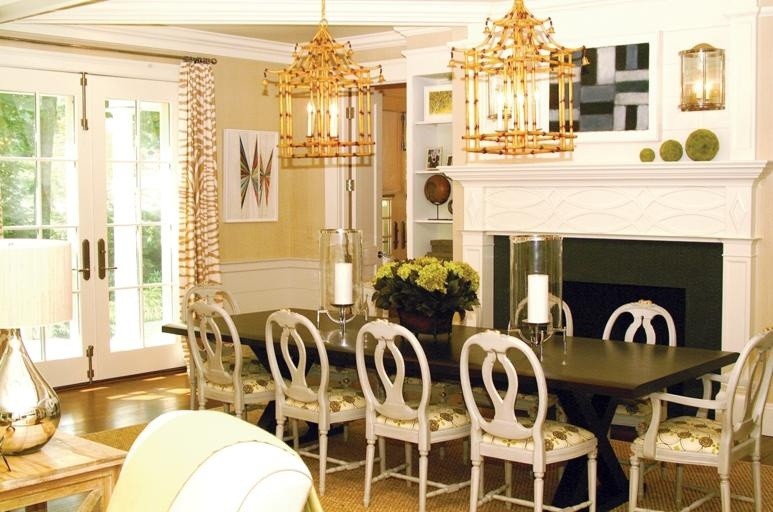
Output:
[396,306,454,341]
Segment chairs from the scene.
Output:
[260,307,386,495]
[104,405,320,512]
[352,317,484,512]
[305,360,380,444]
[180,278,270,412]
[183,298,299,449]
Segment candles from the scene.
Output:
[331,261,354,306]
[524,272,550,325]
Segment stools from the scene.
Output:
[389,305,481,462]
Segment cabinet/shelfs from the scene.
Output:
[404,73,454,257]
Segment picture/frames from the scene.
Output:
[539,28,661,143]
[423,86,453,122]
[220,129,282,224]
[424,143,443,171]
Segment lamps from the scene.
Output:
[0,236,74,455]
[446,1,591,157]
[677,43,724,112]
[259,2,387,160]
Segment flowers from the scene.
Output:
[372,256,481,312]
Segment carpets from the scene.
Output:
[78,417,773,512]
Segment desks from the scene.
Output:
[160,307,739,512]
[0,427,126,512]
